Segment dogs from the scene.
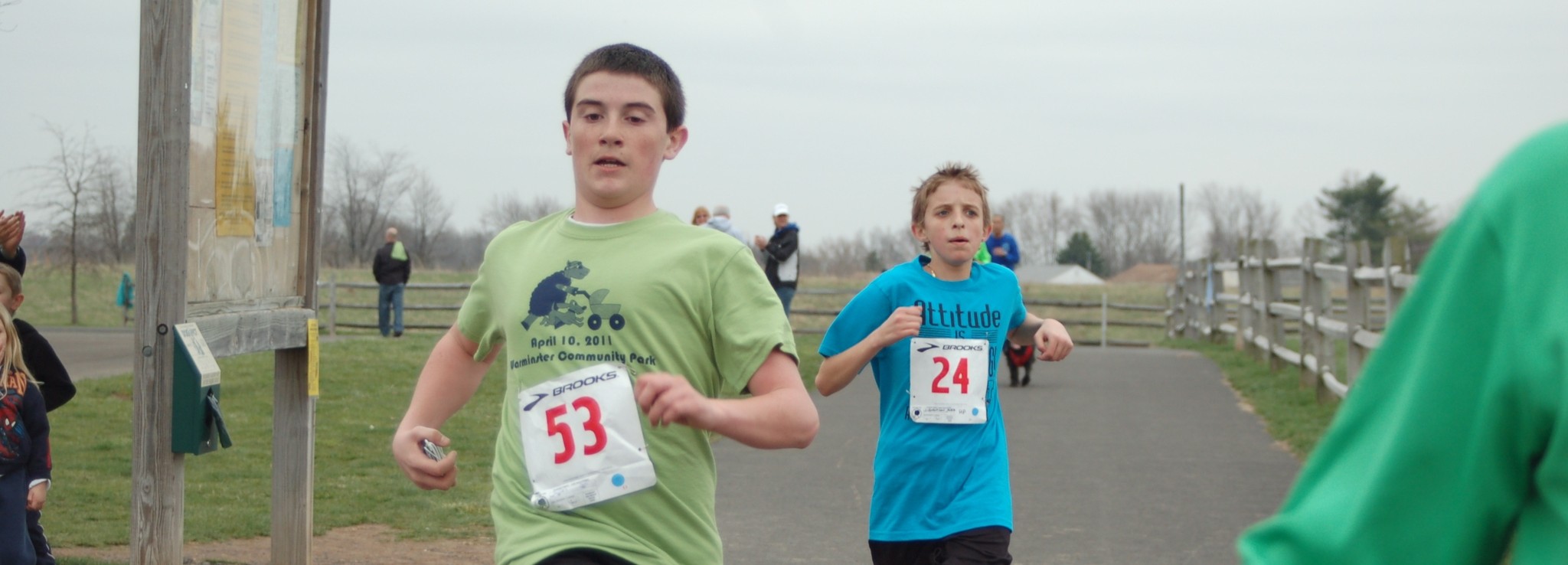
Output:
[1003,339,1036,386]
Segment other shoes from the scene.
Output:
[394,331,402,337]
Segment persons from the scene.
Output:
[0,209,77,565]
[1235,123,1568,564]
[393,45,819,565]
[755,205,801,319]
[692,205,750,246]
[373,228,411,337]
[973,215,1020,272]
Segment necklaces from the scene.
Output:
[927,264,934,277]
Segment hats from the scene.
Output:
[0,243,26,276]
[771,203,788,217]
